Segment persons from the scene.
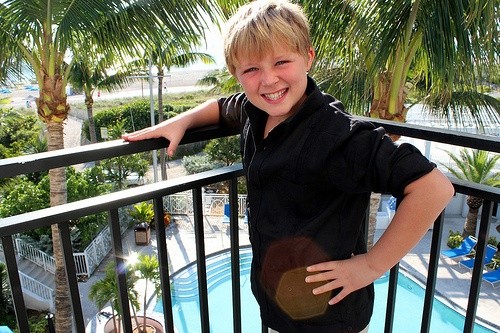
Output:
[121,0,455,333]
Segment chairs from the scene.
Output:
[222,204,230,224]
[482,266,500,289]
[440,235,478,261]
[458,243,498,273]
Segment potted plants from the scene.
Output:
[88,255,175,333]
[127,202,155,245]
[438,146,500,237]
